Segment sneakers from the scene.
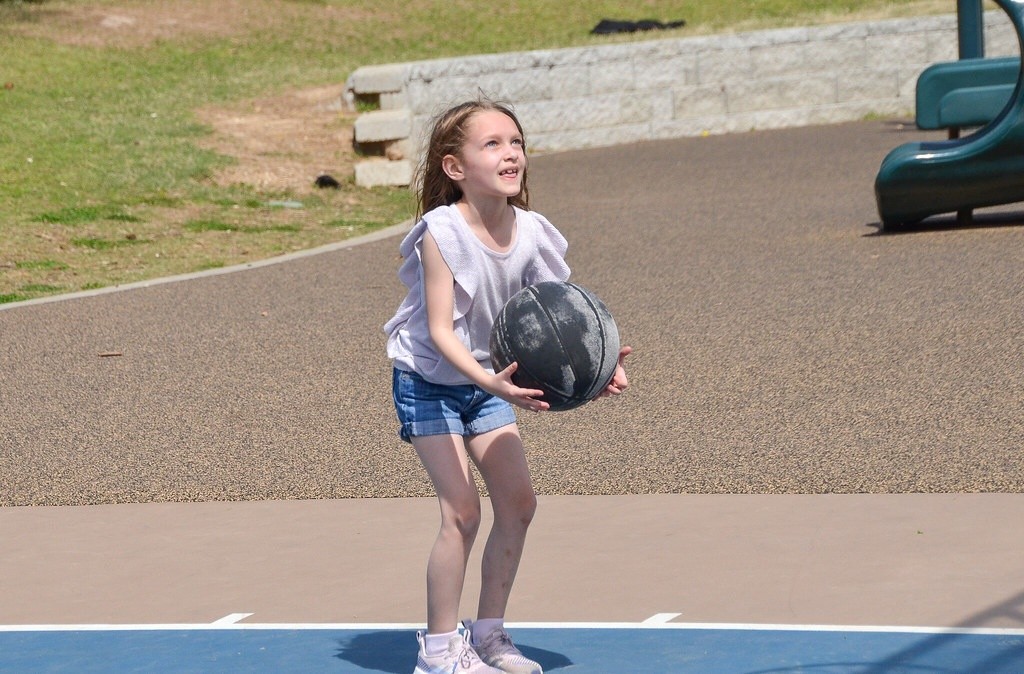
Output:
[414,630,543,674]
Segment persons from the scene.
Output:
[383,87,631,674]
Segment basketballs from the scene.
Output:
[489,281,620,412]
